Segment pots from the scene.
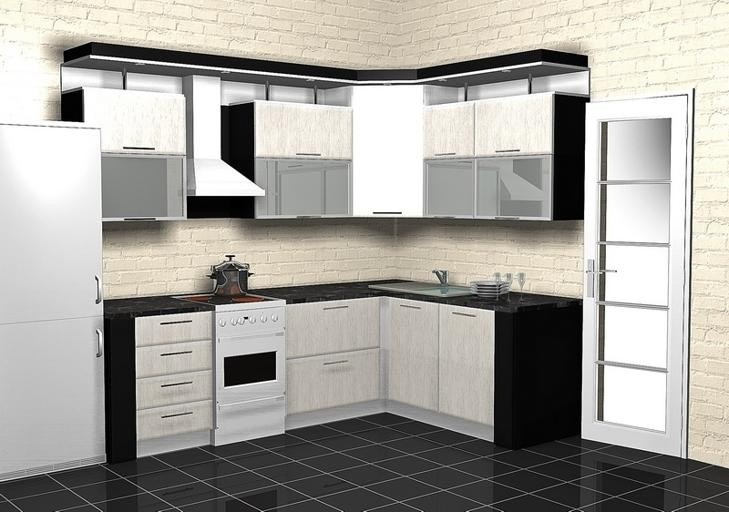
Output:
[207,255,255,298]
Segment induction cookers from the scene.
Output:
[170,292,287,338]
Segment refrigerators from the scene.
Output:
[0,122,109,489]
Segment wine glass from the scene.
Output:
[494,272,502,302]
[517,272,527,304]
[504,273,514,303]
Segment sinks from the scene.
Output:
[415,284,475,299]
[366,278,447,294]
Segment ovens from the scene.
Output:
[210,335,286,448]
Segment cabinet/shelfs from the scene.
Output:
[388,297,495,425]
[352,84,423,219]
[133,309,214,442]
[254,99,352,217]
[83,89,186,223]
[422,93,553,223]
[285,296,380,417]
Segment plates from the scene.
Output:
[470,280,510,298]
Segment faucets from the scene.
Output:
[431,267,449,284]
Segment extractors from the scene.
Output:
[180,74,268,197]
